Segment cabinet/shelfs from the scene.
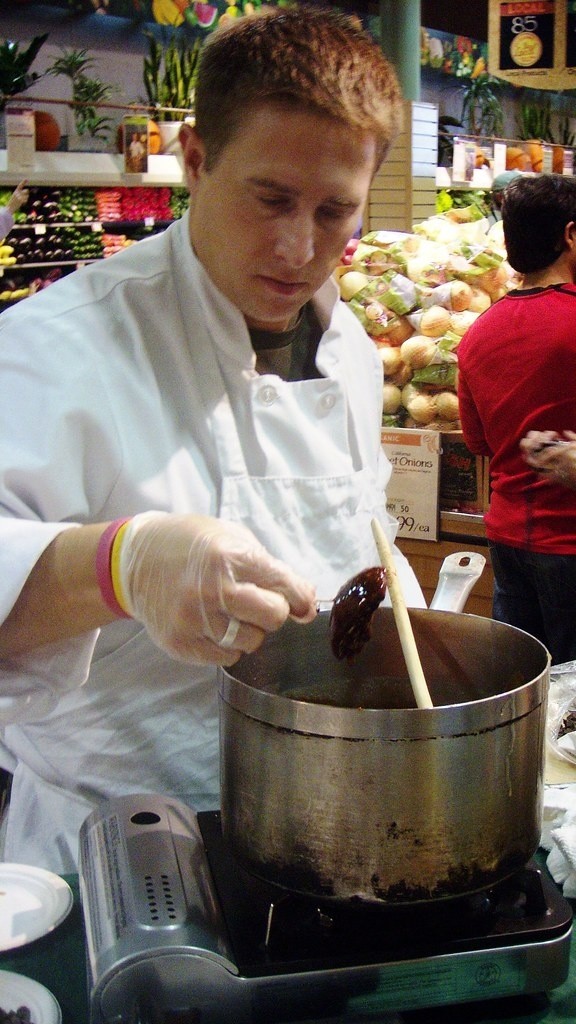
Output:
[0,149,190,313]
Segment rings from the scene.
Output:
[219,620,239,649]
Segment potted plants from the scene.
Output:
[142,22,194,155]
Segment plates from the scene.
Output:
[0,970,63,1024]
[0,863,74,952]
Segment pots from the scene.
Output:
[216,551,551,912]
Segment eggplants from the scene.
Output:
[1,187,75,295]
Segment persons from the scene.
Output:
[517,428,576,482]
[0,3,428,882]
[0,178,30,243]
[456,172,575,664]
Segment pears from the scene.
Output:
[339,260,510,426]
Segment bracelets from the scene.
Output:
[96,516,139,620]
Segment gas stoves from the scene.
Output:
[79,791,573,1024]
